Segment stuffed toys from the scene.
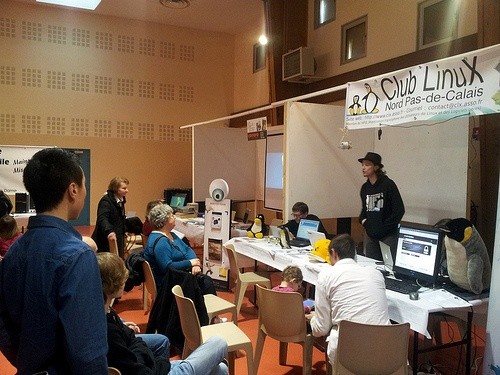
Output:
[307,239,332,265]
[280,227,291,248]
[246,214,267,239]
[438,218,492,293]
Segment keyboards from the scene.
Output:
[385,276,421,294]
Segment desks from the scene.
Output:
[222,237,490,375]
[172,214,248,249]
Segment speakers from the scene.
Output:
[15,193,30,213]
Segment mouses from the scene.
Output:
[410,291,418,300]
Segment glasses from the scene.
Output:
[291,213,304,217]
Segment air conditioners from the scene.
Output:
[281,47,315,81]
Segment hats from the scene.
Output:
[358,152,384,169]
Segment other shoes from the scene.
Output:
[212,316,227,324]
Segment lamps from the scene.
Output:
[338,141,352,149]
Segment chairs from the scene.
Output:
[106,211,414,375]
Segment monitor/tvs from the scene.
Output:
[392,223,444,287]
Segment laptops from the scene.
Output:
[295,219,327,245]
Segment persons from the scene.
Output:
[305,233,392,366]
[91,177,129,306]
[143,200,228,324]
[95,252,230,375]
[82,235,170,361]
[284,201,328,239]
[358,152,406,266]
[0,147,109,375]
[271,266,314,334]
